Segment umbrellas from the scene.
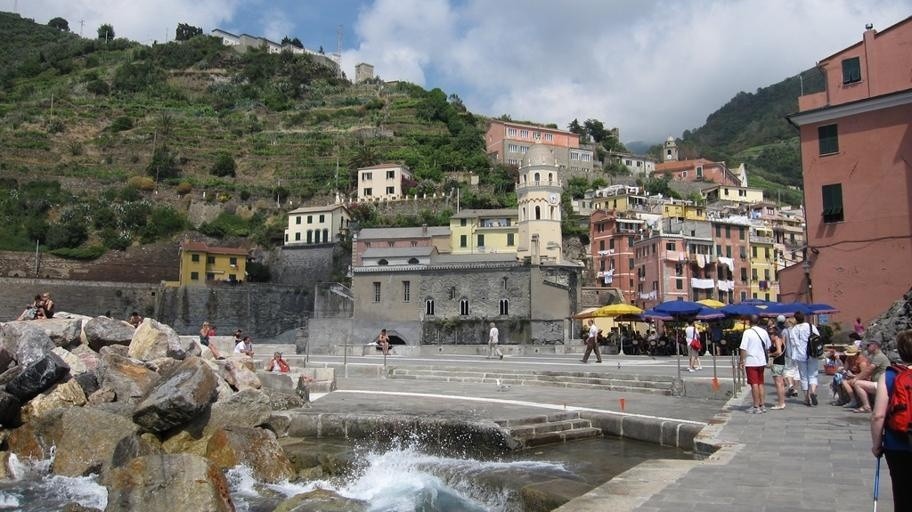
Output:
[571,296,840,332]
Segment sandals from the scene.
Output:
[832,398,872,412]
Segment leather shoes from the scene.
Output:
[597,361,601,362]
[580,360,587,363]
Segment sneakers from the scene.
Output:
[745,406,762,414]
[687,366,702,372]
[804,400,811,406]
[789,390,798,397]
[775,402,786,407]
[810,393,817,405]
[760,405,767,412]
[770,405,784,410]
[784,385,793,395]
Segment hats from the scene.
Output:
[866,338,882,346]
[844,344,859,357]
[777,314,785,323]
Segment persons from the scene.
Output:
[758,315,800,410]
[685,320,702,372]
[581,320,740,356]
[740,314,771,413]
[234,329,243,345]
[485,320,503,361]
[579,320,602,363]
[199,321,226,360]
[233,335,255,358]
[128,310,143,328]
[376,328,392,355]
[264,351,291,373]
[36,292,56,320]
[789,312,820,405]
[17,294,44,321]
[817,317,889,413]
[869,329,911,510]
[210,322,217,336]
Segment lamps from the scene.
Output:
[801,257,813,276]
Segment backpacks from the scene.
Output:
[886,364,912,433]
[806,322,823,359]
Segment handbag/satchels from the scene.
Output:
[766,356,774,368]
[690,327,700,351]
